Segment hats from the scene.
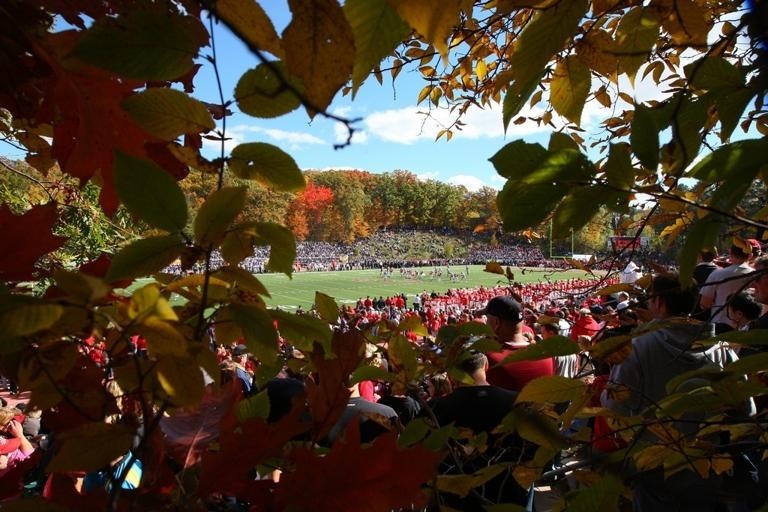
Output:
[474,295,526,322]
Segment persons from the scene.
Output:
[2,225,767,512]
